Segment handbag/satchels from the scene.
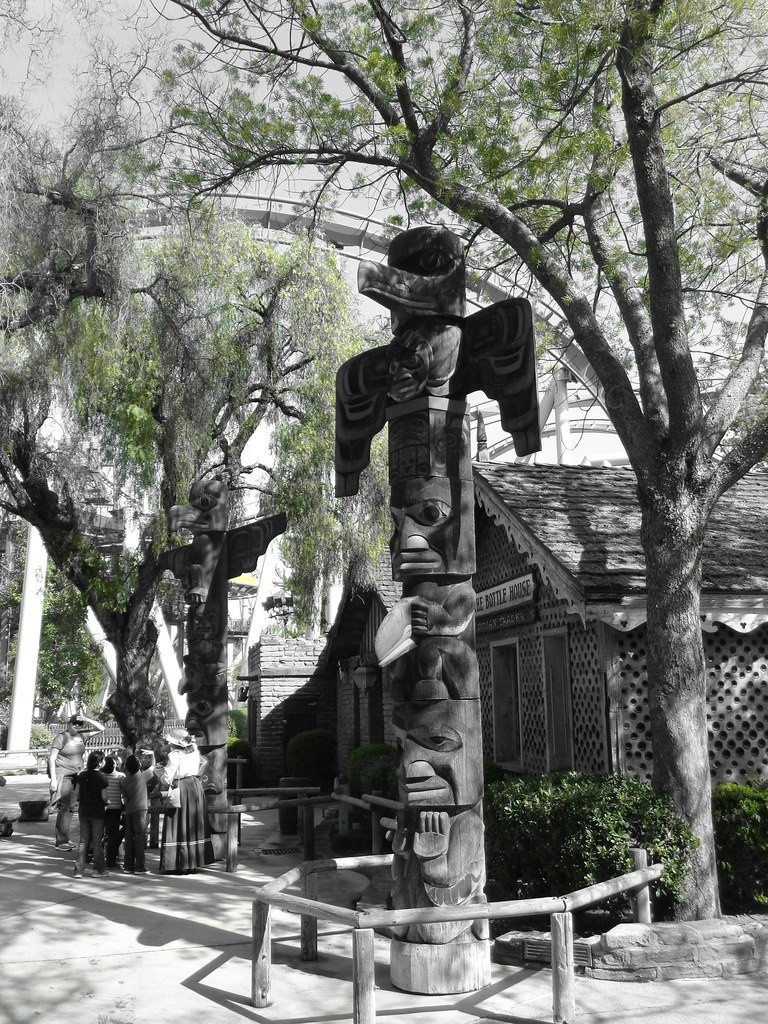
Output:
[166,788,181,808]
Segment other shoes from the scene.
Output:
[134,870,150,875]
[123,868,135,873]
[85,857,94,864]
[55,844,72,851]
[106,864,122,869]
[92,870,110,877]
[72,871,82,877]
[63,841,76,848]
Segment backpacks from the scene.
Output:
[46,732,84,778]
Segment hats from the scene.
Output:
[166,729,196,747]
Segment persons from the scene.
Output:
[83,753,125,873]
[72,751,113,879]
[121,748,157,876]
[45,712,106,851]
[159,729,217,876]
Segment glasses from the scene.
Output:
[71,721,83,726]
